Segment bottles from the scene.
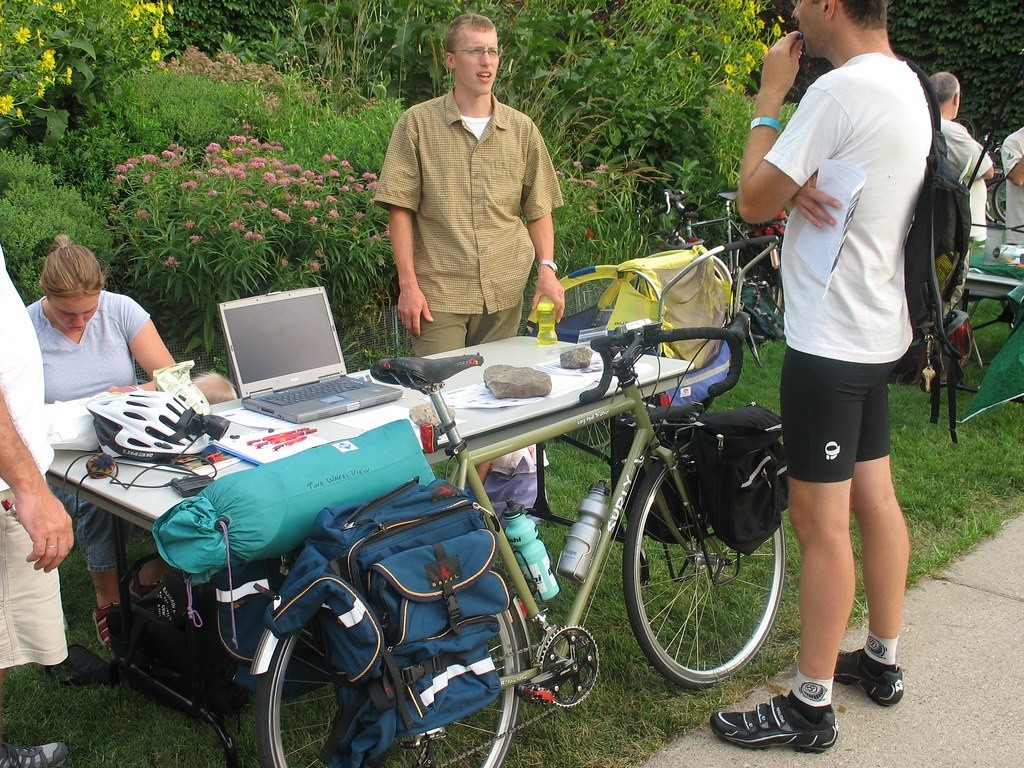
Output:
[1,498,16,517]
[984,220,1006,262]
[557,480,610,582]
[537,296,558,345]
[502,499,560,602]
[720,256,729,269]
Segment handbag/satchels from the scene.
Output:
[693,404,791,557]
[612,406,696,544]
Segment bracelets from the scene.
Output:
[133,385,144,391]
[750,116,780,131]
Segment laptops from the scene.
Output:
[216,285,403,423]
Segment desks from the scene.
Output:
[941,271,1024,404]
[43,335,695,767]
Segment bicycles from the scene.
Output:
[979,132,1006,224]
[250,316,788,768]
[652,187,784,320]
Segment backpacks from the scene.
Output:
[43,476,516,768]
[892,49,973,444]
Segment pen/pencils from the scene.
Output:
[272,435,307,451]
[246,427,318,448]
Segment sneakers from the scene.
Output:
[92,600,125,646]
[0,742,68,768]
[710,694,839,753]
[833,648,904,707]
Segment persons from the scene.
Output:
[0,246,75,768]
[928,73,993,318]
[25,234,175,646]
[1001,128,1024,246]
[710,0,932,753]
[374,12,564,359]
[194,374,236,403]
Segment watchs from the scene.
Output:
[537,260,558,275]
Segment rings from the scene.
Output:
[45,545,58,548]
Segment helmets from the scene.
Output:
[86,391,209,464]
[886,310,972,386]
